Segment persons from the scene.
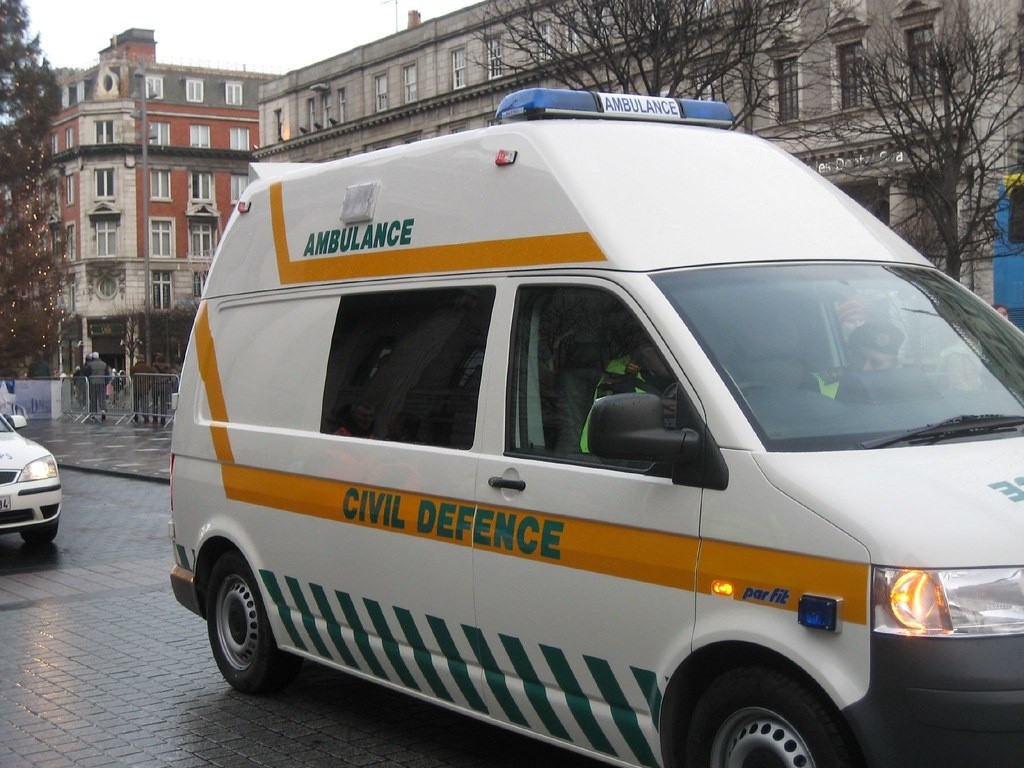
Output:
[579,330,673,456]
[334,403,420,445]
[16,350,183,425]
[803,303,1009,406]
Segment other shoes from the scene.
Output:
[102,412,106,420]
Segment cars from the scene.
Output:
[0,410,60,545]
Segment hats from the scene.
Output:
[86,353,92,359]
[847,320,905,356]
[91,352,99,359]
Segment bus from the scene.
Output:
[992,170,1024,334]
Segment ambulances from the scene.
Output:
[168,73,1024,767]
